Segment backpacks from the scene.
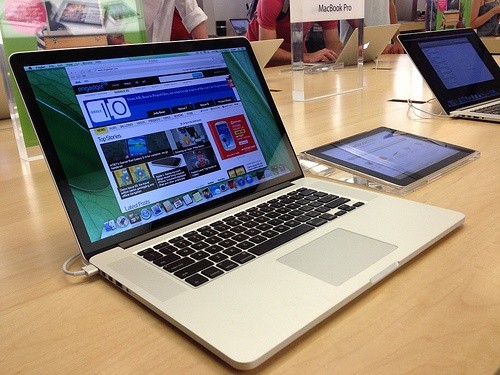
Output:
[246,0,289,23]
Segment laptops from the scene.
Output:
[9,36,465,369]
[230,19,250,35]
[397,28,500,122]
[303,24,401,66]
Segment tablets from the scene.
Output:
[300,126,479,190]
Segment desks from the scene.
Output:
[0,55,500,375]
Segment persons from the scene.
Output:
[245,0,347,65]
[139,0,208,42]
[471,0,500,36]
[340,0,405,53]
[183,131,210,169]
